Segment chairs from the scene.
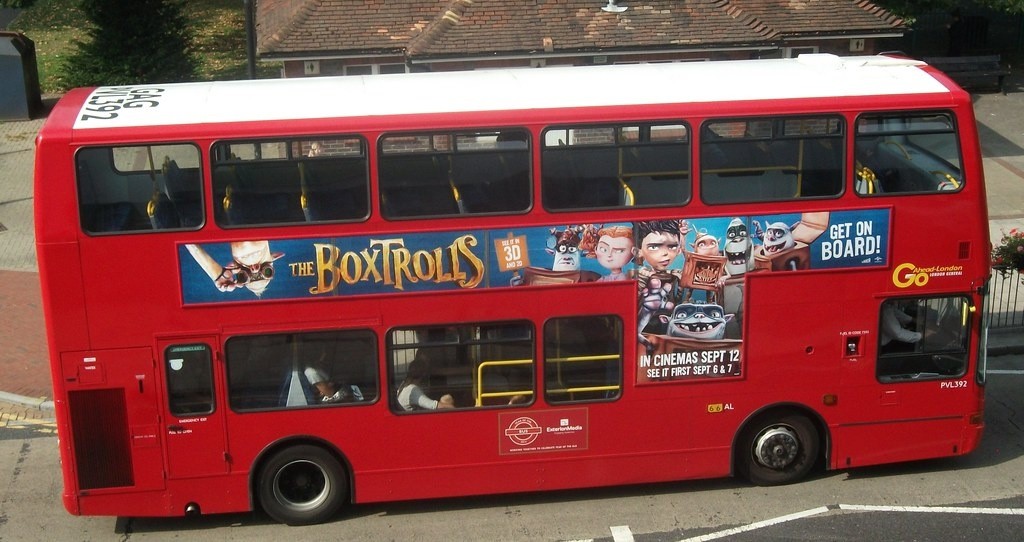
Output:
[225,185,245,216]
[297,155,325,222]
[147,201,177,229]
[631,142,884,201]
[429,154,471,214]
[152,181,202,226]
[229,153,254,195]
[223,197,246,227]
[162,163,203,215]
[164,156,202,203]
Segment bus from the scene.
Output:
[35,53,992,526]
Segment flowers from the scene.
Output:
[992,228,1024,285]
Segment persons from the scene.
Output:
[297,338,364,404]
[877,303,937,353]
[394,360,456,412]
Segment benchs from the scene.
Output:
[77,159,132,233]
[876,140,957,193]
